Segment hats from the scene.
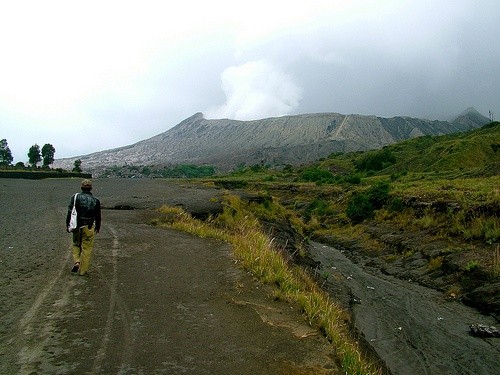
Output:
[80,179,93,188]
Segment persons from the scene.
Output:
[65,179,102,276]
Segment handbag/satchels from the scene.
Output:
[68,192,78,232]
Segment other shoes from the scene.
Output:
[71,262,80,272]
